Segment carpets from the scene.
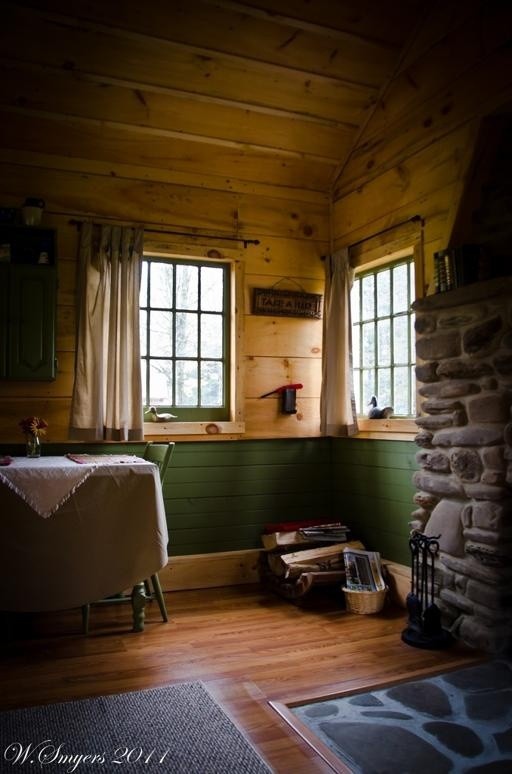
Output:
[0,679,278,774]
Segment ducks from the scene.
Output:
[367,395,394,420]
[144,406,178,422]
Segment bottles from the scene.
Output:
[431,245,465,292]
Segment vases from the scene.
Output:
[25,436,41,458]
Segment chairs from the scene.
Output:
[82,440,178,632]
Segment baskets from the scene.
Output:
[341,582,389,615]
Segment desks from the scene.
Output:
[0,451,160,641]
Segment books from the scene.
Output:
[258,516,385,592]
[433,241,484,293]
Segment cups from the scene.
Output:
[282,388,298,415]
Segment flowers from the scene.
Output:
[18,416,49,455]
